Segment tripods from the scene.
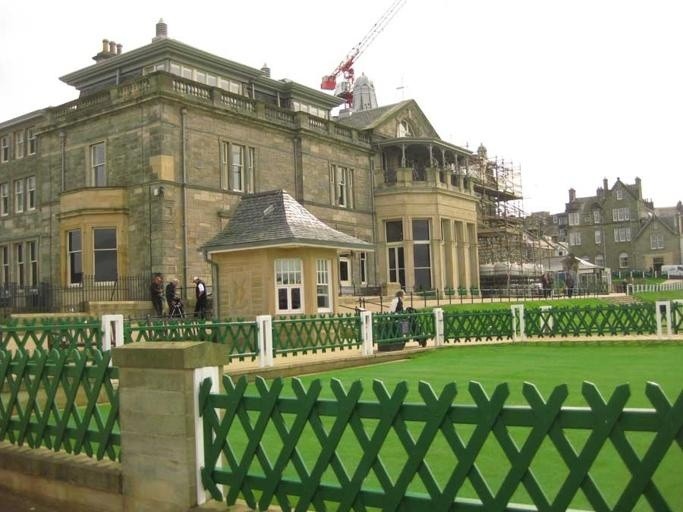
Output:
[170,304,191,319]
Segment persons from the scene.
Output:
[165,279,181,317]
[388,289,406,315]
[151,276,163,318]
[542,273,574,299]
[191,277,208,322]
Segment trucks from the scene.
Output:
[659,264,682,280]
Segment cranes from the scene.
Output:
[321,0,412,103]
